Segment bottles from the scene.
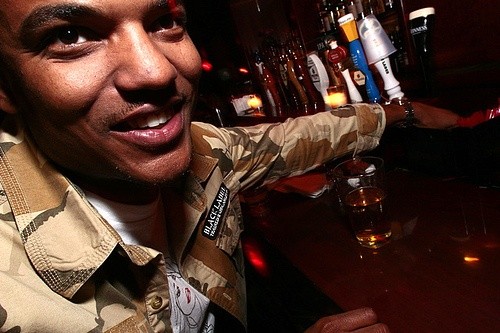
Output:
[318,0,404,36]
[250,37,330,109]
[209,106,230,129]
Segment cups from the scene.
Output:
[248,94,264,111]
[407,6,436,61]
[326,84,348,110]
[358,15,397,64]
[331,155,392,245]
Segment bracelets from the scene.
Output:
[390,98,415,128]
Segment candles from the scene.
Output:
[327,91,348,109]
[248,97,263,109]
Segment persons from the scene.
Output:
[0,0,464,333]
